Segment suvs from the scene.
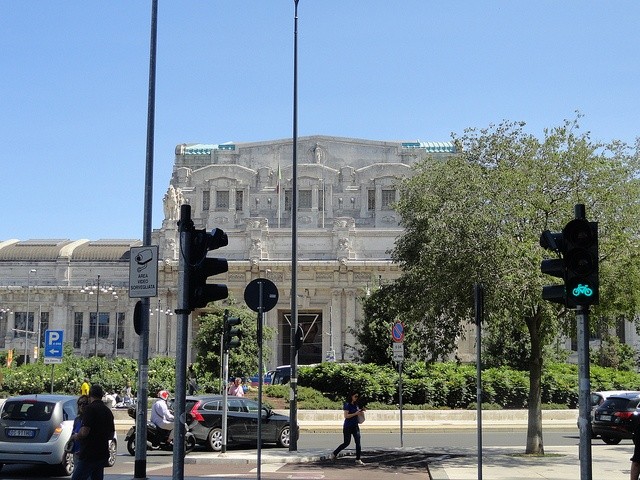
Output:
[591,393,640,444]
[168,395,299,452]
[1,394,117,477]
[577,391,640,437]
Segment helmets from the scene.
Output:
[158,390,173,401]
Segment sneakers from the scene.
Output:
[331,454,336,461]
[355,459,366,465]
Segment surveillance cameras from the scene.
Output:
[134,249,153,272]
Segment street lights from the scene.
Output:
[24,269,37,366]
[79,274,119,359]
[149,299,174,355]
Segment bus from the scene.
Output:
[266,363,349,387]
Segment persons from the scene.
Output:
[71,385,115,480]
[150,390,174,446]
[123,382,134,407]
[115,391,126,408]
[81,378,90,397]
[65,395,90,475]
[330,391,366,467]
[630,431,640,480]
[228,378,244,397]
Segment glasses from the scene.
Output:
[78,401,89,405]
[354,394,359,398]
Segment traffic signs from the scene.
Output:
[43,330,63,364]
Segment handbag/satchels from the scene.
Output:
[357,405,365,424]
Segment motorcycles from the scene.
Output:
[124,405,197,454]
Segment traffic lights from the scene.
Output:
[564,220,599,304]
[540,230,578,309]
[189,228,228,309]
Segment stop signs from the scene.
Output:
[392,321,405,342]
